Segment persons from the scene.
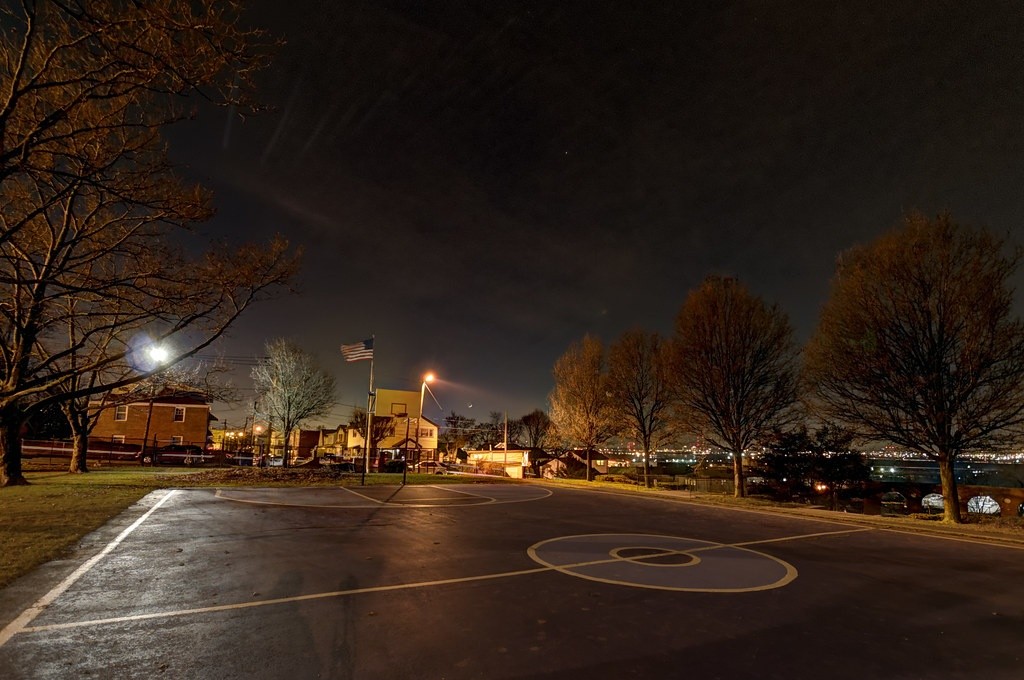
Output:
[908,491,916,502]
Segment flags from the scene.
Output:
[340,334,374,361]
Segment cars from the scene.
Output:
[408,459,450,475]
[136,445,208,468]
[257,452,381,471]
[383,459,406,474]
[403,459,417,472]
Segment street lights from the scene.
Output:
[139,346,168,466]
[415,370,434,450]
